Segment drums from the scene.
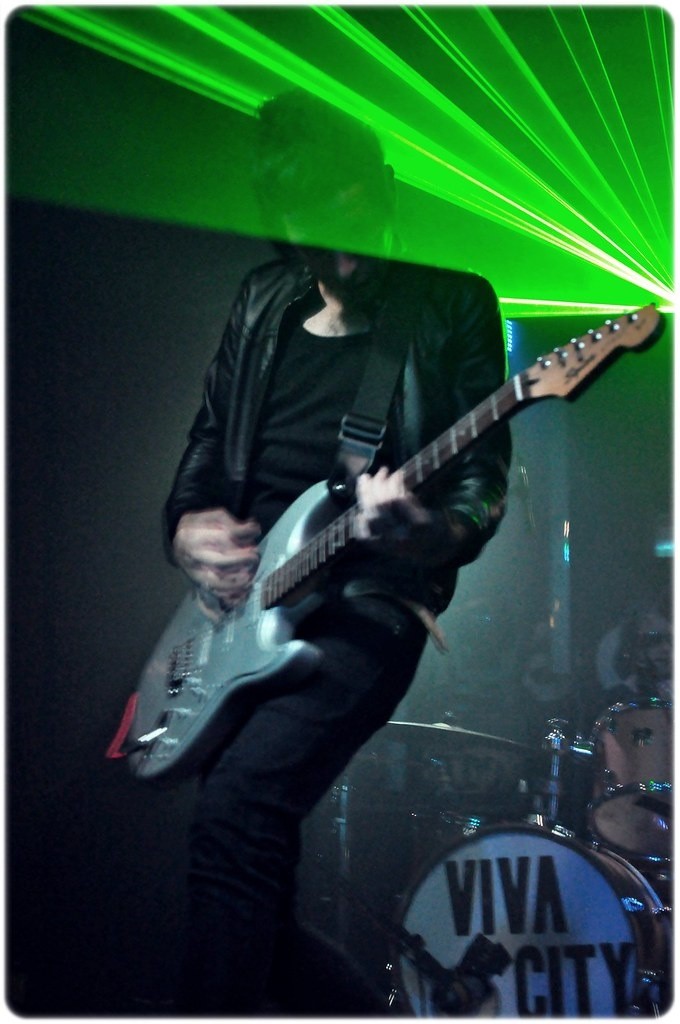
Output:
[594,695,671,799]
[584,790,671,866]
[392,823,672,1016]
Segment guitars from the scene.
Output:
[115,295,671,796]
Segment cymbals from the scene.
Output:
[384,719,531,753]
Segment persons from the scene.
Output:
[441,601,674,798]
[162,93,513,1018]
